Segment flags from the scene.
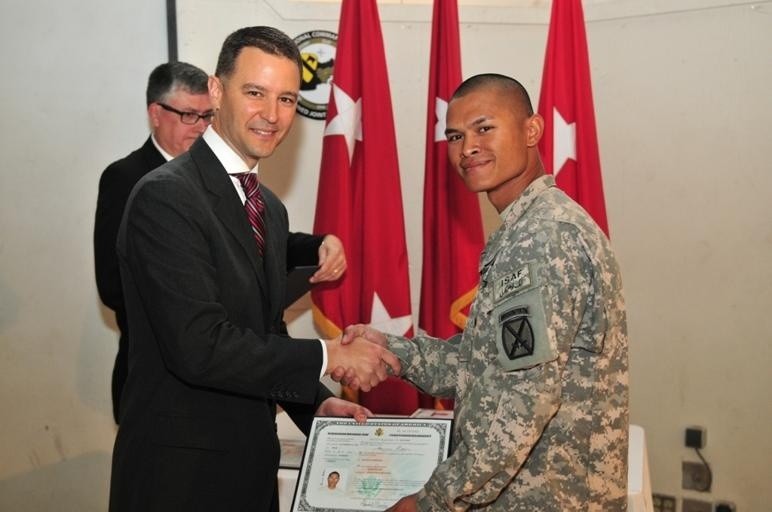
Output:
[311,1,418,418]
[419,2,485,407]
[537,1,609,237]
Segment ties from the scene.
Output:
[229,171,268,258]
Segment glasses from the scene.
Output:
[156,103,212,125]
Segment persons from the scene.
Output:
[107,27,401,510]
[330,73,629,512]
[319,472,344,494]
[94,61,347,423]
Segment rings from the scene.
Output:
[334,269,339,273]
